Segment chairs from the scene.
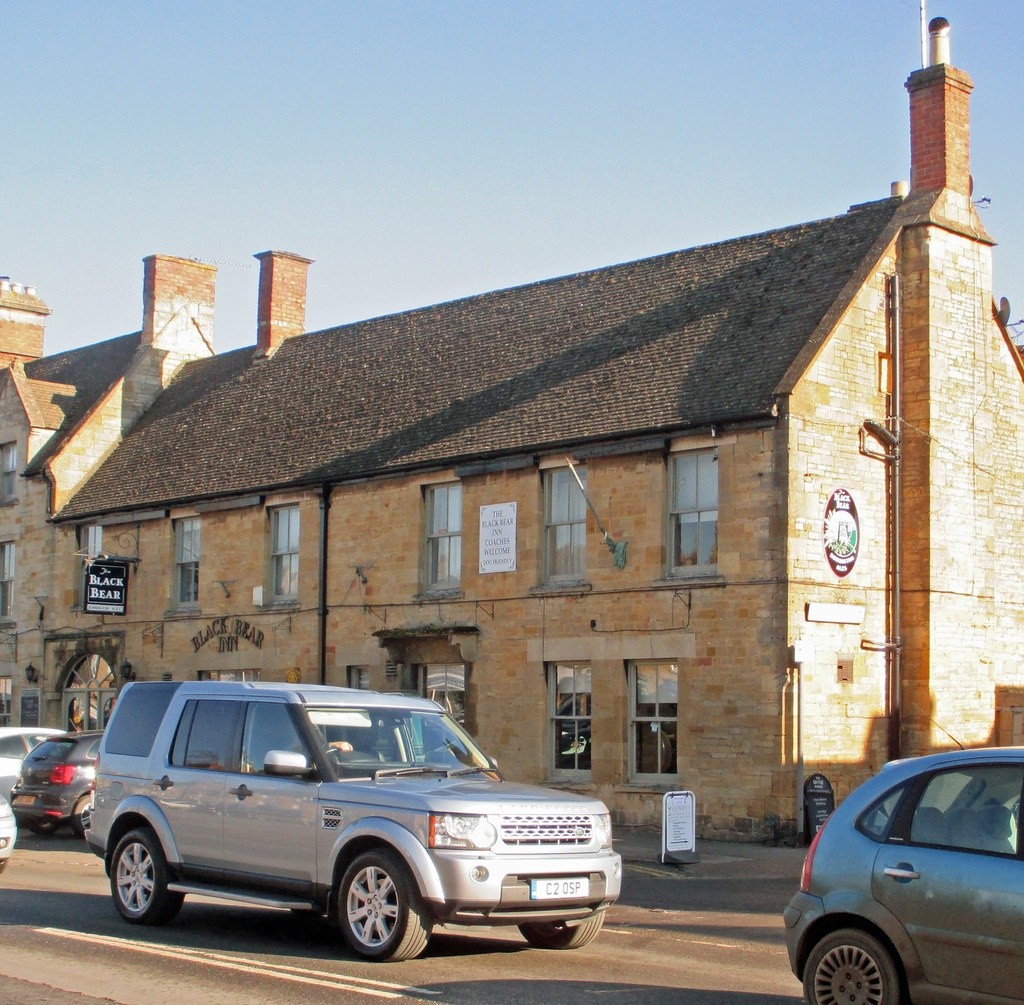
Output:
[910,807,947,846]
[960,804,1015,856]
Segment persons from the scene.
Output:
[328,741,353,752]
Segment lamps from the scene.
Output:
[212,580,237,599]
[347,564,375,584]
[25,662,38,684]
[28,595,50,622]
[120,658,136,681]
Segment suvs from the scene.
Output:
[85,678,623,964]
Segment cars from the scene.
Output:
[781,745,1024,1005]
[10,729,108,836]
[0,726,72,802]
